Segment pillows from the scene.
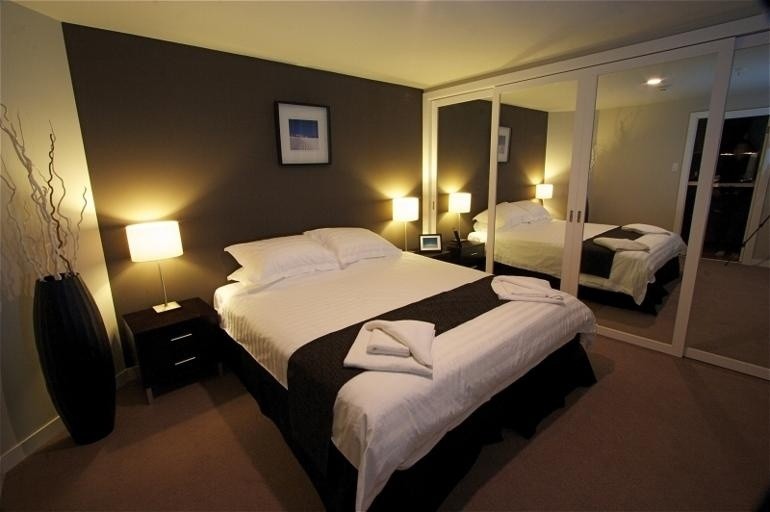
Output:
[226,261,291,295]
[473,198,550,236]
[217,235,343,282]
[301,227,412,265]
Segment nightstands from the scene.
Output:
[403,245,452,261]
[124,298,225,404]
[440,238,486,273]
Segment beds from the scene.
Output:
[469,220,684,315]
[210,249,602,507]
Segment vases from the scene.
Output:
[31,271,117,446]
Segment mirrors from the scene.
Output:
[663,44,770,366]
[436,95,493,273]
[491,77,580,297]
[571,50,721,345]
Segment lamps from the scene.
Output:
[446,190,475,242]
[390,195,421,253]
[122,219,184,315]
[534,183,554,208]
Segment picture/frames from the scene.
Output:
[272,99,333,167]
[491,122,511,163]
[451,230,462,248]
[418,233,443,252]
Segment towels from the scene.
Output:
[621,221,671,237]
[591,235,651,254]
[490,273,567,309]
[342,317,436,379]
